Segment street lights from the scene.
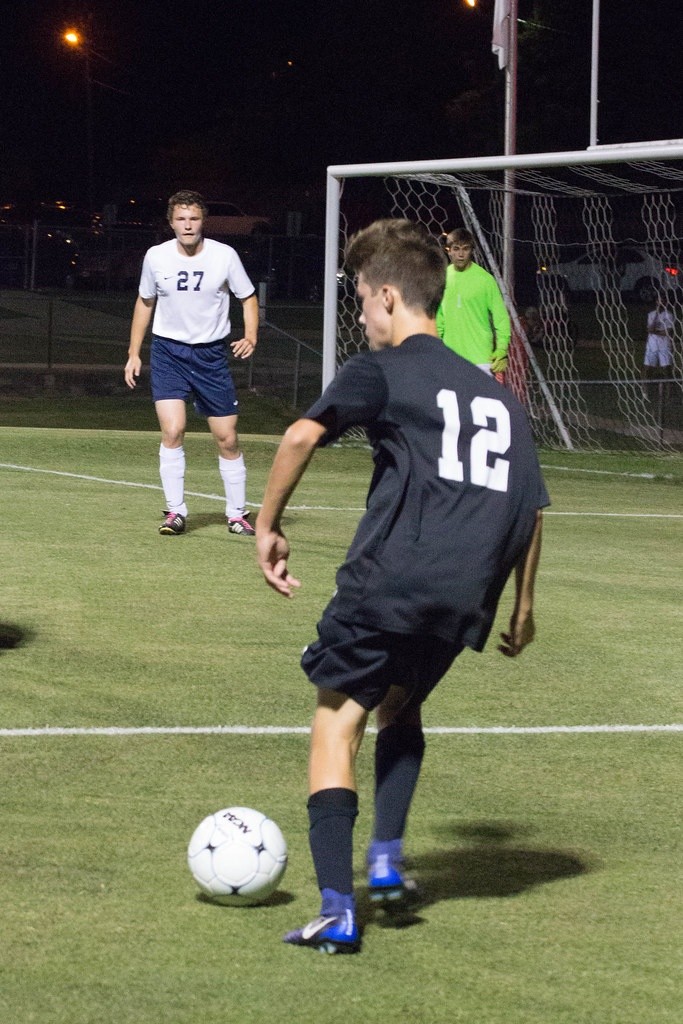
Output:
[64,29,97,215]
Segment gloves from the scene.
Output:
[489,349,509,372]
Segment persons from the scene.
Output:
[638,294,674,405]
[254,219,551,954]
[125,189,259,535]
[436,228,510,384]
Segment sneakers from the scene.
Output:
[158,509,185,534]
[366,855,418,902]
[228,511,255,536]
[282,909,358,954]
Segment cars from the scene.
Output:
[248,234,356,307]
[510,272,573,349]
[204,199,275,247]
[535,243,683,306]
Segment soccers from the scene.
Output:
[186,805,289,907]
